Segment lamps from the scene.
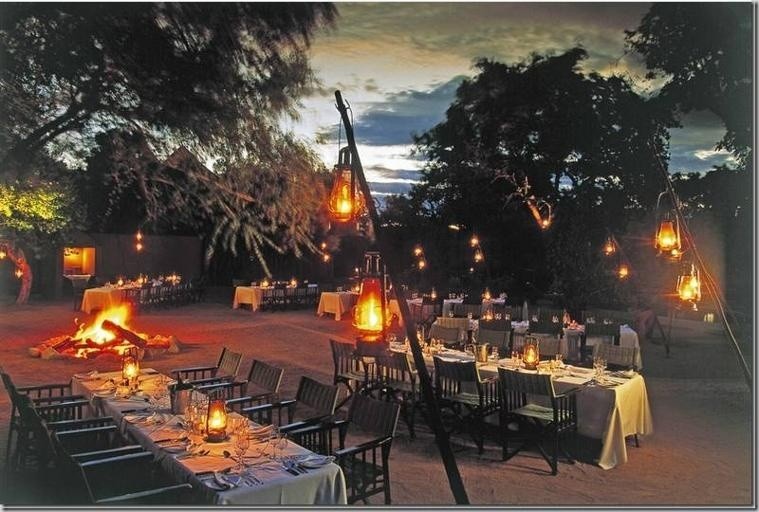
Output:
[675,257,701,311]
[654,187,682,256]
[327,147,364,220]
[352,249,394,359]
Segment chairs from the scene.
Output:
[51,430,194,508]
[399,284,636,368]
[175,347,244,387]
[73,274,208,310]
[232,284,319,311]
[0,362,85,468]
[200,360,285,423]
[242,378,341,433]
[286,392,402,508]
[17,393,115,473]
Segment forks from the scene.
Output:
[282,461,309,477]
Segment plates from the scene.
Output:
[284,455,336,469]
[124,414,154,426]
[204,473,242,492]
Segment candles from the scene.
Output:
[521,336,540,371]
[204,392,230,447]
[121,347,141,386]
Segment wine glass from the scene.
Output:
[536,353,565,378]
[270,429,288,463]
[592,357,608,379]
[487,346,520,369]
[231,417,251,471]
[185,400,208,437]
